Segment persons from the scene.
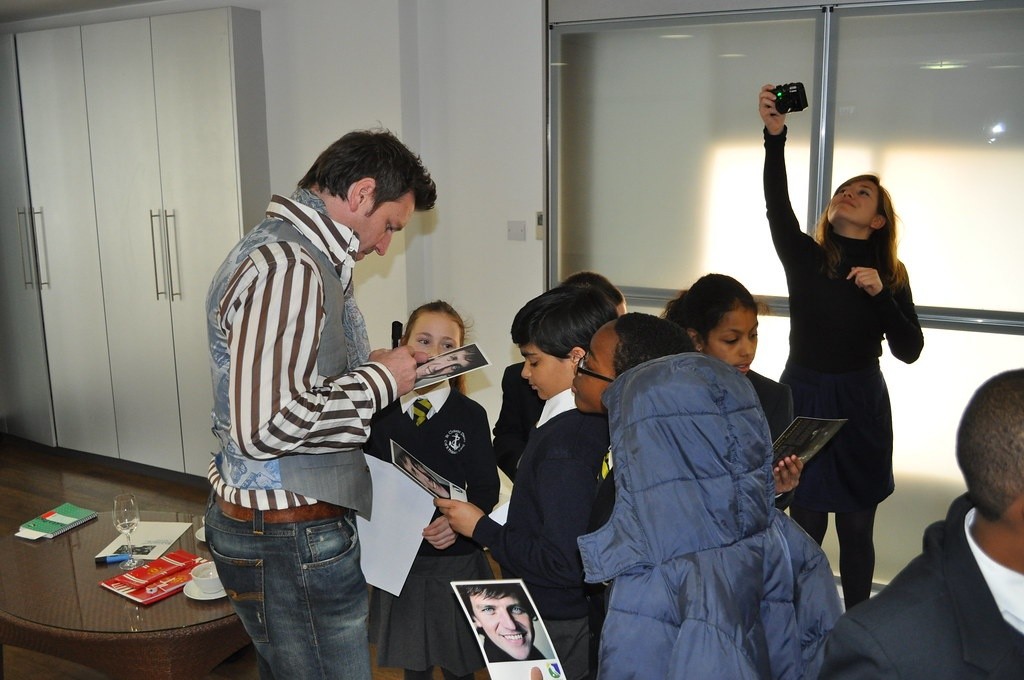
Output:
[759,84,924,612]
[393,442,450,499]
[819,369,1023,680]
[362,299,501,680]
[490,271,847,680]
[205,131,437,680]
[456,583,547,663]
[415,344,488,384]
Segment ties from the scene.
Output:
[413,399,433,427]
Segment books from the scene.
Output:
[101,548,208,605]
[14,502,99,540]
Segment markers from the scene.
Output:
[94,553,129,565]
[392,321,403,349]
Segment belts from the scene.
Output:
[211,491,353,523]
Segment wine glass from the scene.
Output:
[112,493,144,571]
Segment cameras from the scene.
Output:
[766,82,808,116]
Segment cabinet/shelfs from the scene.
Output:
[0,25,120,460]
[79,7,272,479]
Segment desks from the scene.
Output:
[0,511,253,680]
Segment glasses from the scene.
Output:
[576,350,613,383]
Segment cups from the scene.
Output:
[191,561,223,594]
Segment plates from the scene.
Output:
[195,526,206,542]
[183,579,228,601]
